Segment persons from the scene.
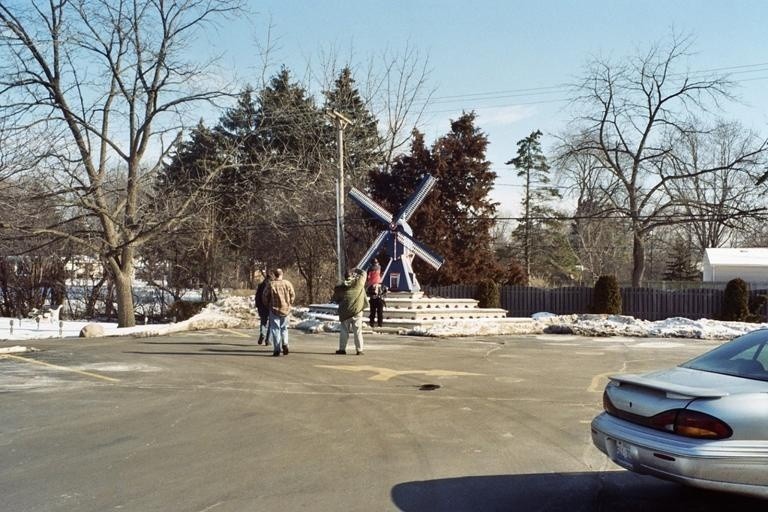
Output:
[333,257,388,355]
[255,269,296,356]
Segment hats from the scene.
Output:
[370,258,379,264]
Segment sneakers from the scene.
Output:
[273,345,289,356]
[335,350,364,355]
[258,334,271,345]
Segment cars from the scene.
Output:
[591,327,768,497]
